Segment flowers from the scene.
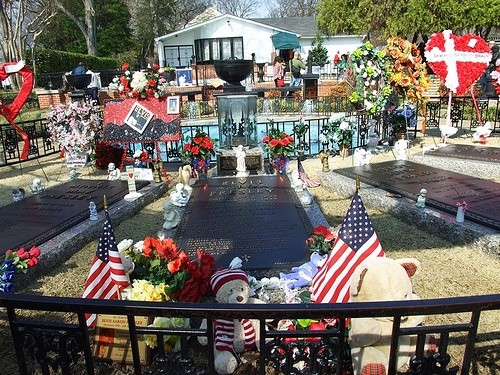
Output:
[117,235,216,353]
[306,225,336,256]
[43,97,123,169]
[349,29,500,132]
[0,245,41,293]
[108,60,167,100]
[275,290,351,372]
[260,118,298,158]
[178,127,223,165]
[132,149,152,168]
[320,112,356,147]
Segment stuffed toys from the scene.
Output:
[197,267,266,375]
[347,256,426,375]
[279,251,328,289]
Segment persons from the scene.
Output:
[272,56,287,88]
[74,61,100,101]
[415,189,427,208]
[175,183,187,204]
[235,145,247,172]
[288,51,306,87]
[9,163,117,222]
[162,201,175,229]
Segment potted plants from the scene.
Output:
[163,67,176,83]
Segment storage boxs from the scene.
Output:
[93,313,153,366]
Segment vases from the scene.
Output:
[395,130,405,141]
[272,156,290,175]
[193,159,211,181]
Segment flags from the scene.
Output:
[312,191,386,327]
[82,211,129,328]
